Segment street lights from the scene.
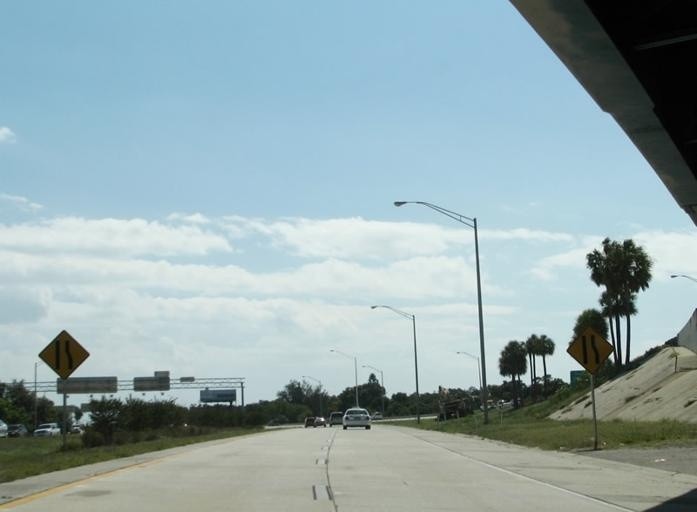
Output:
[330,200,490,425]
[671,273,697,285]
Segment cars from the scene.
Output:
[304,407,373,429]
[1,419,89,438]
